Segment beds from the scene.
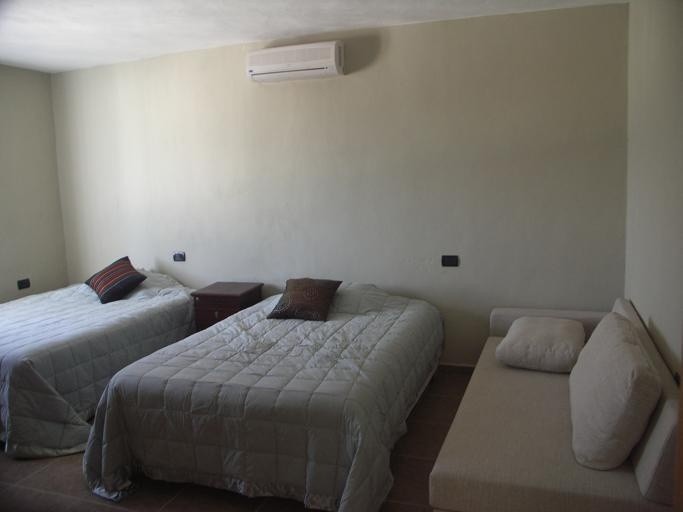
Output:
[428,297,683,512]
[81,277,444,512]
[0,265,196,459]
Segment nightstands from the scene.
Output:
[191,282,264,334]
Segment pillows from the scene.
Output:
[85,257,145,304]
[497,318,586,372]
[567,310,662,471]
[268,280,342,322]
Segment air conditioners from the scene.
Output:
[247,39,344,82]
